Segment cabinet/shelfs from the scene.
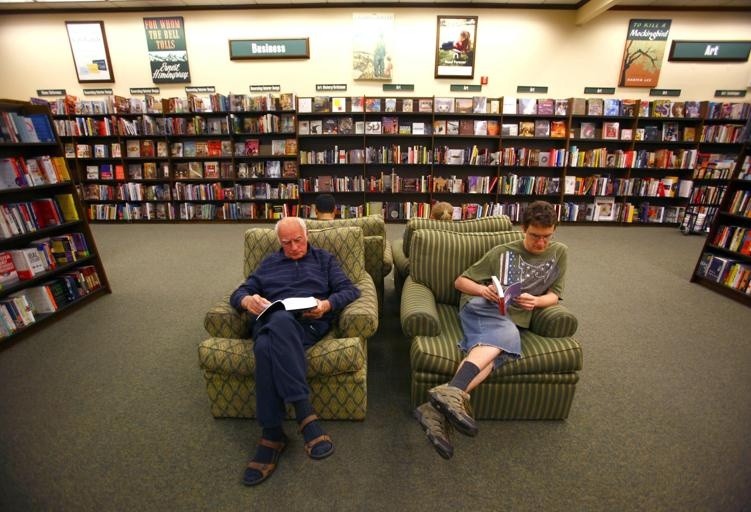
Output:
[364,95,434,224]
[294,95,367,220]
[685,100,751,228]
[116,114,177,225]
[229,112,301,224]
[69,114,133,223]
[690,102,751,309]
[1,98,112,353]
[431,95,503,221]
[496,95,575,226]
[559,97,641,227]
[164,114,239,223]
[620,99,709,228]
[51,115,87,225]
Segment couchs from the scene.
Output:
[391,215,514,301]
[400,230,584,422]
[197,226,379,421]
[305,214,392,319]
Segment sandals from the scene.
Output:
[297,412,337,461]
[241,429,289,487]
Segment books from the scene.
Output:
[0,233,90,288]
[501,173,561,196]
[298,144,365,162]
[335,198,363,217]
[502,199,528,224]
[0,156,72,191]
[500,119,566,137]
[298,116,363,134]
[627,200,688,223]
[700,124,745,144]
[641,100,702,118]
[738,155,751,180]
[0,193,79,239]
[636,121,695,142]
[709,224,751,257]
[58,138,298,155]
[435,96,500,114]
[502,146,565,167]
[365,116,432,134]
[433,174,497,193]
[364,201,430,218]
[254,294,320,322]
[365,143,431,164]
[682,150,739,235]
[365,167,431,193]
[697,251,751,295]
[298,97,363,112]
[46,90,296,113]
[0,264,102,340]
[452,201,498,220]
[52,114,296,136]
[565,175,629,196]
[433,119,499,135]
[299,201,317,218]
[564,197,624,223]
[634,147,696,170]
[578,121,630,140]
[727,188,750,217]
[487,274,524,316]
[433,143,498,166]
[85,203,297,220]
[570,145,632,169]
[76,181,299,201]
[503,96,570,114]
[299,175,363,191]
[84,160,299,180]
[571,98,635,116]
[366,98,432,112]
[708,102,749,119]
[551,202,562,223]
[1,110,56,144]
[630,175,692,199]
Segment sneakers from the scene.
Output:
[426,383,478,438]
[411,399,457,461]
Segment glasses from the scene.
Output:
[526,230,553,241]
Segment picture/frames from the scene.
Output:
[228,38,310,59]
[65,20,115,84]
[668,40,751,62]
[434,14,478,80]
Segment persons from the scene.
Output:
[430,202,453,220]
[439,30,471,53]
[229,217,361,487]
[416,199,570,460]
[311,195,336,221]
